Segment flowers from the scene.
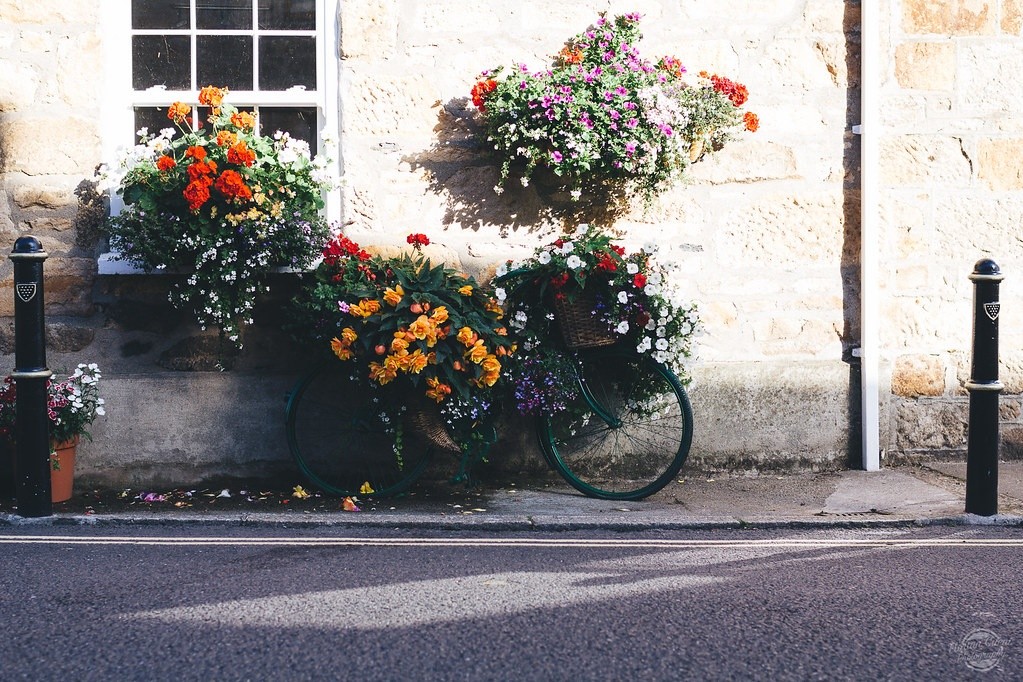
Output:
[489,219,702,440]
[291,231,582,481]
[103,84,331,373]
[0,362,101,470]
[467,0,761,205]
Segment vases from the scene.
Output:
[9,435,78,503]
[96,182,341,277]
[536,165,607,210]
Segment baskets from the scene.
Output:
[396,395,462,455]
[551,284,622,350]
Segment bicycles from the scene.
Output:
[283,331,695,502]
